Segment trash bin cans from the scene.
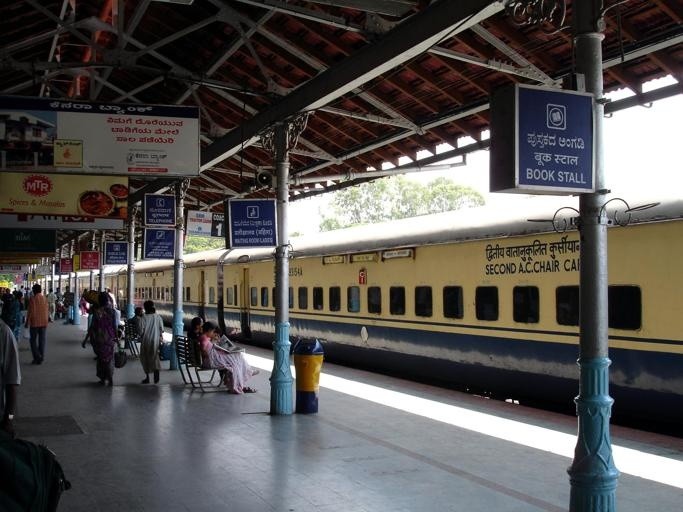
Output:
[293,337,325,414]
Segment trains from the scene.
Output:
[55,198,681,442]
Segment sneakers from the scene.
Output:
[242,386,257,393]
[142,371,160,384]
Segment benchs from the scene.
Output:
[175,335,232,393]
[124,319,143,358]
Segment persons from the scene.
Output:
[80,285,260,394]
[0,285,75,438]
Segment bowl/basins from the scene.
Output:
[77,182,128,218]
